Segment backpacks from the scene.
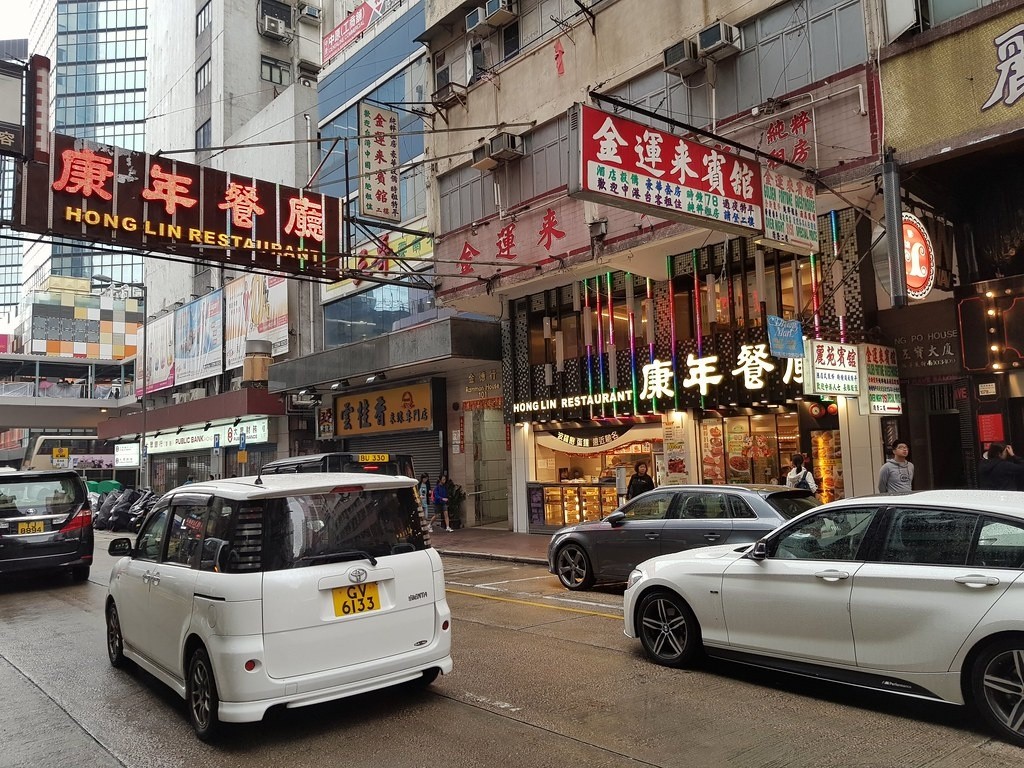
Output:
[795,470,810,494]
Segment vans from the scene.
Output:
[102,473,452,741]
[261,451,416,478]
[0,469,93,583]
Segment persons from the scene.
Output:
[416,472,433,529]
[182,474,236,486]
[778,467,788,488]
[980,441,1024,494]
[55,377,120,398]
[427,474,454,533]
[785,453,819,496]
[625,461,655,502]
[876,438,915,497]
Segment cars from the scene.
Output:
[547,481,823,591]
[623,488,1023,739]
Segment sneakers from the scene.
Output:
[427,524,433,532]
[446,528,454,532]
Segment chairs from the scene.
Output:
[883,514,937,564]
[713,498,733,518]
[681,497,708,519]
[934,520,958,562]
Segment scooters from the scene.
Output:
[84,480,159,533]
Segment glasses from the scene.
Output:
[896,445,909,450]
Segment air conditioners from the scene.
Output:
[489,133,524,160]
[485,0,518,26]
[661,38,707,77]
[262,15,286,38]
[299,76,317,90]
[470,143,497,171]
[302,6,319,19]
[695,22,743,62]
[466,7,495,40]
